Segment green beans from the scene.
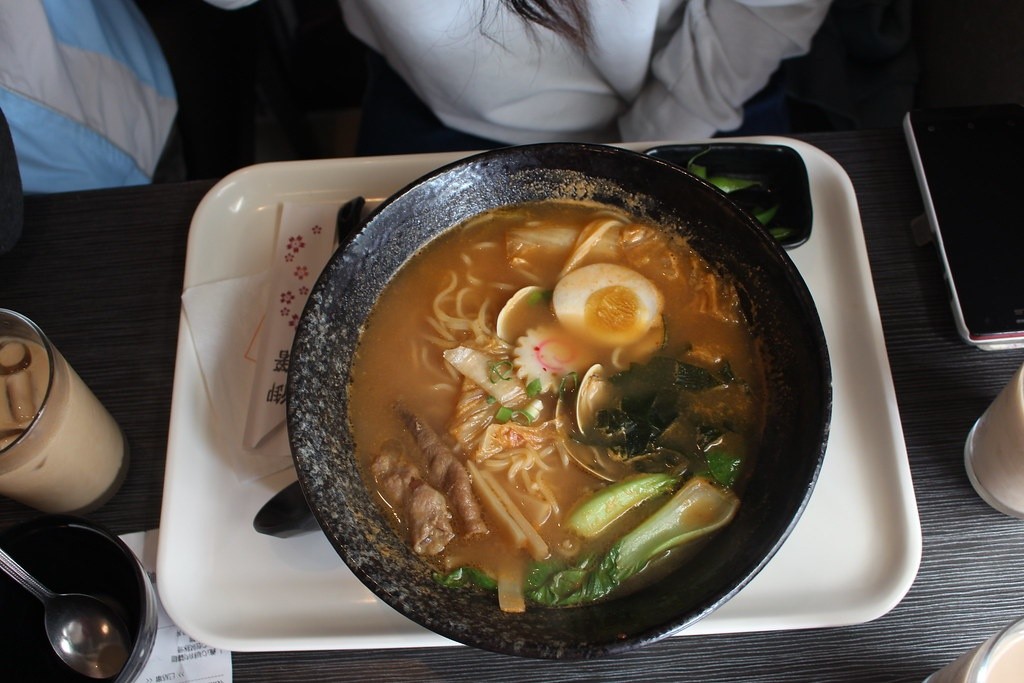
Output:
[689,164,795,240]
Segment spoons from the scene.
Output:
[0,545,130,680]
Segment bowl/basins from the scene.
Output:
[285,141,833,656]
[0,521,158,683]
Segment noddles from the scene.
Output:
[343,197,764,610]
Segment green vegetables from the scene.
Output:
[432,447,754,607]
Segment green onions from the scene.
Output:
[482,292,578,428]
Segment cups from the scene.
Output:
[0,308,129,515]
[962,357,1024,525]
[920,612,1024,683]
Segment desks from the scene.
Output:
[1,128,1024,683]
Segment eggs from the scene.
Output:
[552,262,664,344]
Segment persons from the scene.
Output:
[200,0,834,156]
[0,0,186,194]
